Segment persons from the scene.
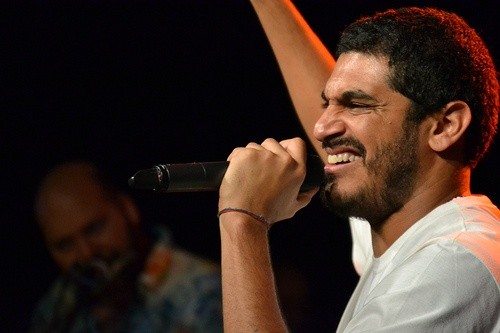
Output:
[28,160,224,333]
[218,0,500,333]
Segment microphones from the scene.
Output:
[127,154,326,197]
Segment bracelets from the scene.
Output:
[216,207,270,230]
